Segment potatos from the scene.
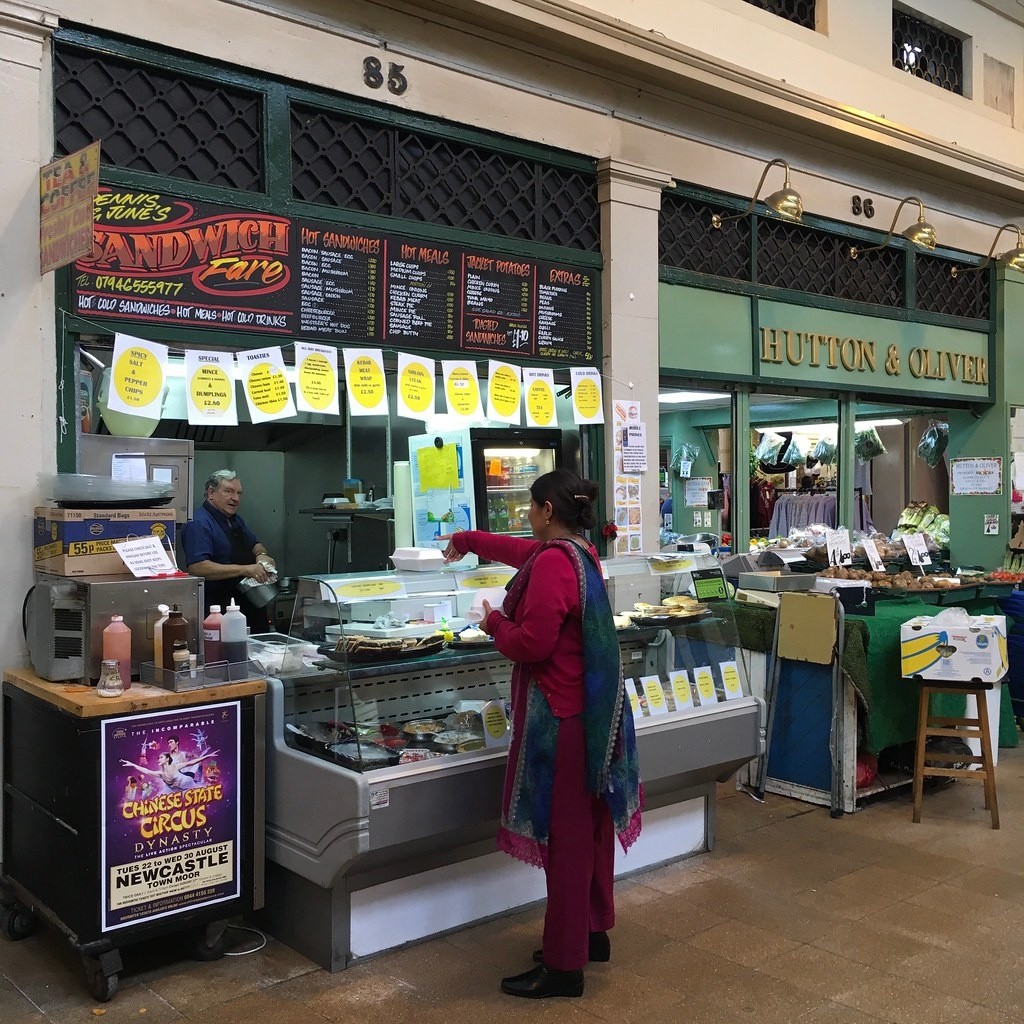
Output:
[768,529,907,557]
[814,566,952,590]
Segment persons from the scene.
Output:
[436,469,646,997]
[181,470,276,635]
[721,489,729,519]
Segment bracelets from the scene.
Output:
[255,550,267,556]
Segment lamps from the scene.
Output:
[851,196,937,259]
[710,157,803,230]
[754,417,913,434]
[951,223,1024,279]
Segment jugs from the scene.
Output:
[343,479,362,503]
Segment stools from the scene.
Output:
[910,675,1001,830]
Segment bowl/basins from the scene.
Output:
[247,631,313,674]
[389,547,446,572]
[96,401,168,437]
[292,721,403,773]
[676,532,717,545]
[401,719,486,753]
[320,492,378,510]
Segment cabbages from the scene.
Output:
[925,513,950,549]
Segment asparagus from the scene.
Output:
[1003,550,1024,575]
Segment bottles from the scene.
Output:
[162,603,191,691]
[154,603,173,682]
[485,455,539,531]
[102,615,131,689]
[203,605,225,678]
[221,597,249,682]
[172,641,191,687]
[96,659,124,698]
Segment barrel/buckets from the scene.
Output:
[239,573,280,610]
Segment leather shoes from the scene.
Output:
[533,931,610,963]
[501,964,585,999]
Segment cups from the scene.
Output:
[354,493,366,504]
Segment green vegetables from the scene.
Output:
[917,426,950,468]
[749,436,838,476]
[854,428,886,462]
[891,501,939,536]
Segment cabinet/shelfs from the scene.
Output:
[264,553,768,972]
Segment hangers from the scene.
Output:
[781,486,861,498]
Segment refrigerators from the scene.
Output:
[408,427,564,566]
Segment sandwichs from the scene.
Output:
[337,634,444,654]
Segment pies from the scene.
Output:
[620,595,709,618]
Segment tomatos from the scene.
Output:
[990,571,1024,583]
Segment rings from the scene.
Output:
[255,574,263,580]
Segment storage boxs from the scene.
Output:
[32,506,177,577]
[899,613,1010,684]
